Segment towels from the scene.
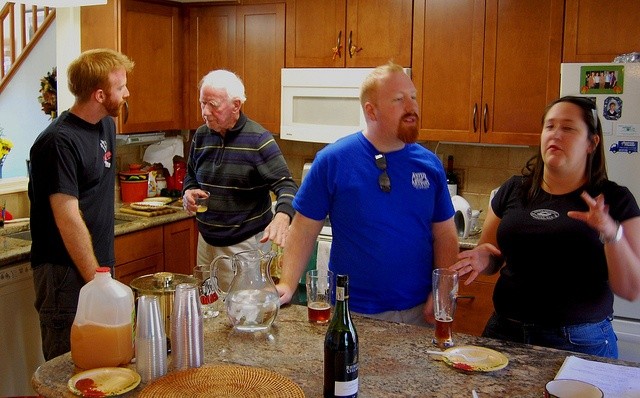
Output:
[297,241,317,304]
[315,240,333,295]
[260,242,285,279]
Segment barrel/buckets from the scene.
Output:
[71,266,135,369]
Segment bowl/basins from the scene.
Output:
[545,378,604,398]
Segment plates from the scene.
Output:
[143,197,173,204]
[442,345,508,372]
[131,202,164,212]
[66,367,141,398]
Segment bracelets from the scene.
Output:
[598,222,624,245]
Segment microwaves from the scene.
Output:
[279,67,411,145]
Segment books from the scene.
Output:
[554,355,640,397]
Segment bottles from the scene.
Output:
[445,154,459,196]
[323,273,359,398]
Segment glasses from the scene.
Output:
[559,96,598,130]
[375,152,391,192]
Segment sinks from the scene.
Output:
[0,214,140,242]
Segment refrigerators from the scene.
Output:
[560,63,639,361]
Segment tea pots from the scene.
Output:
[210,251,279,332]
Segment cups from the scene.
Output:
[305,269,333,322]
[192,191,211,213]
[433,268,459,348]
[171,282,204,369]
[134,295,169,379]
[192,264,220,317]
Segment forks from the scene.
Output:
[427,350,489,363]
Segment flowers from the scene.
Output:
[38,66,58,121]
[0,133,16,164]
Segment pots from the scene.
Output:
[130,272,198,355]
[116,162,149,204]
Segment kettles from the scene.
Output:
[450,195,472,239]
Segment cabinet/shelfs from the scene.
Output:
[284,0,412,68]
[412,0,566,147]
[185,0,285,136]
[561,1,637,64]
[165,214,199,276]
[80,0,185,135]
[450,262,506,336]
[114,225,165,287]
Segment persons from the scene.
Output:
[258,62,460,328]
[28,49,135,361]
[607,102,618,116]
[586,71,617,89]
[181,69,299,294]
[447,96,640,358]
